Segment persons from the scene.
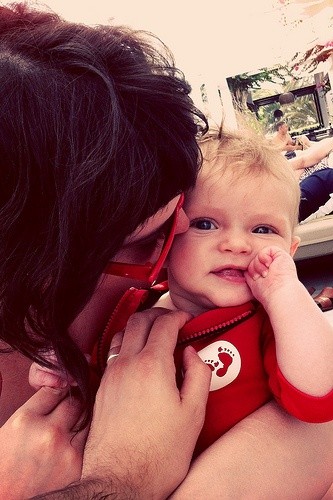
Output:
[271,121,296,148]
[0,307,333,500]
[288,136,333,223]
[0,2,201,432]
[28,131,333,461]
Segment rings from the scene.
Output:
[106,354,119,362]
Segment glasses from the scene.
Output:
[102,193,186,288]
